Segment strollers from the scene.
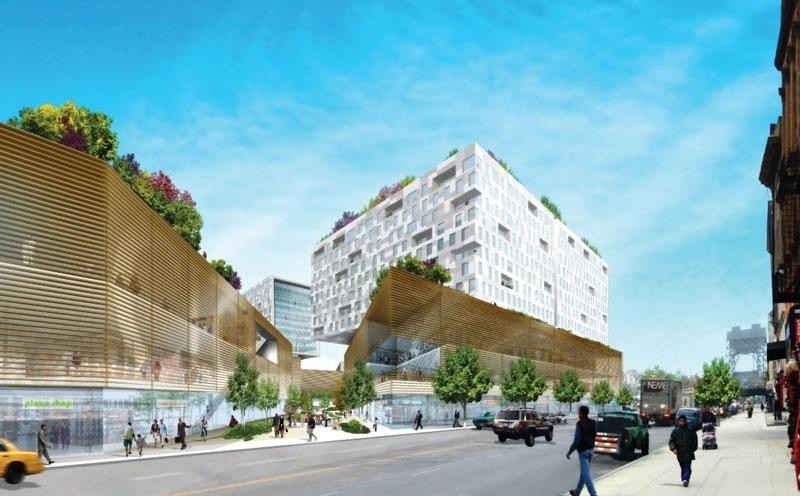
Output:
[699,422,718,450]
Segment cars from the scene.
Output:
[675,408,702,431]
[0,438,45,487]
[543,412,568,425]
[471,411,495,430]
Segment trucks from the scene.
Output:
[640,379,682,426]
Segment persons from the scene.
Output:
[761,402,765,412]
[37,424,56,465]
[453,410,460,427]
[379,352,424,382]
[372,417,378,432]
[746,400,753,418]
[668,415,698,487]
[123,414,237,457]
[272,410,329,442]
[50,421,94,453]
[414,409,423,431]
[701,406,716,430]
[66,348,204,386]
[567,405,599,496]
[114,271,143,297]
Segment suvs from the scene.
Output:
[493,408,553,448]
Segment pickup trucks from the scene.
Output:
[592,412,649,461]
